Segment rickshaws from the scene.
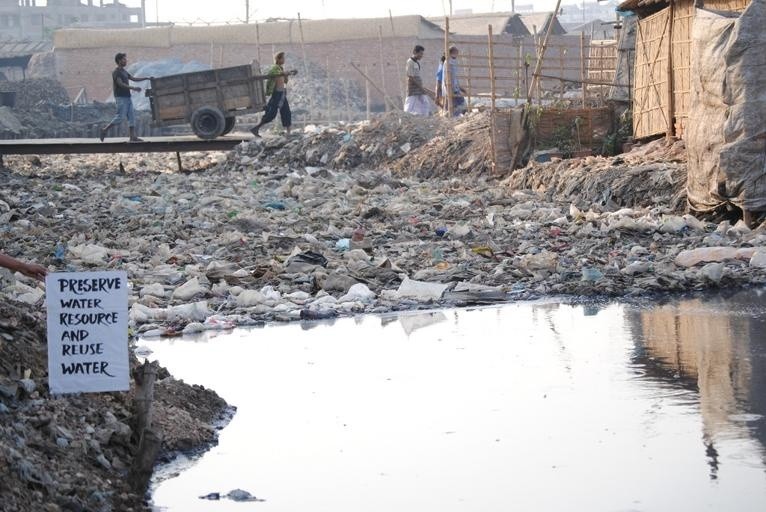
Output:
[144,59,296,139]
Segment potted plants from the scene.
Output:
[537,124,568,162]
[570,116,593,157]
[618,110,633,152]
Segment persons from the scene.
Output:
[250,52,297,137]
[99,53,152,141]
[403,45,468,116]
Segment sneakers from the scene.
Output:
[250,127,261,137]
[130,136,143,141]
[100,128,106,142]
